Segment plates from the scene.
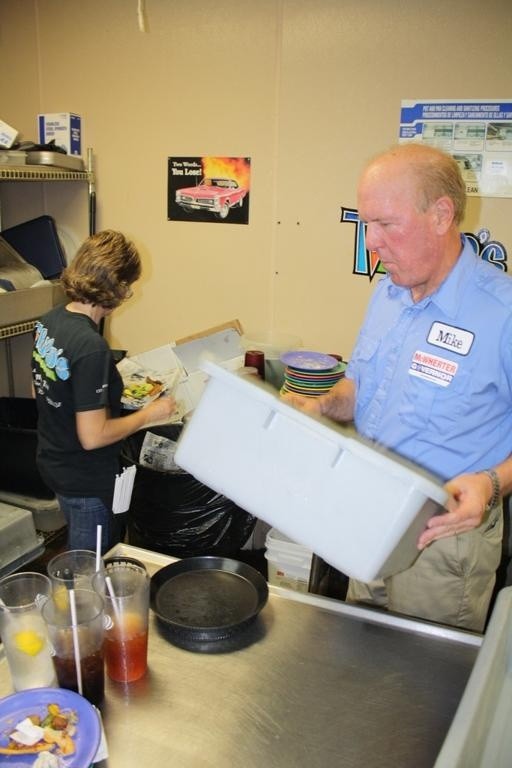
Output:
[2,688,102,767]
[281,352,349,401]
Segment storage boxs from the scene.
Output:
[0,279,65,327]
[0,489,67,581]
[174,361,457,585]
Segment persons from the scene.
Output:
[275,140,511,638]
[29,231,183,554]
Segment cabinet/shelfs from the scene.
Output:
[0,147,96,550]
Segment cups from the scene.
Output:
[46,550,103,593]
[6,151,28,166]
[98,555,145,599]
[0,571,55,691]
[244,351,265,381]
[42,588,106,706]
[93,568,150,683]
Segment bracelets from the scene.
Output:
[478,468,502,511]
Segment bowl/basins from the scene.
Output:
[150,555,270,643]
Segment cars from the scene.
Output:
[174,177,244,220]
